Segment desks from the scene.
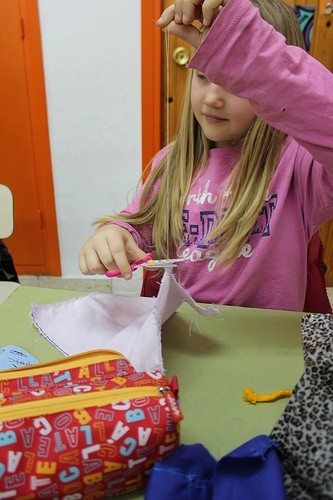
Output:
[0,281,308,500]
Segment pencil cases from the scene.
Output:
[1,349,185,500]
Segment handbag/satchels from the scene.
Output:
[0,349,183,500]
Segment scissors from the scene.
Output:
[105,252,190,278]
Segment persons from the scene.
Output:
[79,0,333,313]
